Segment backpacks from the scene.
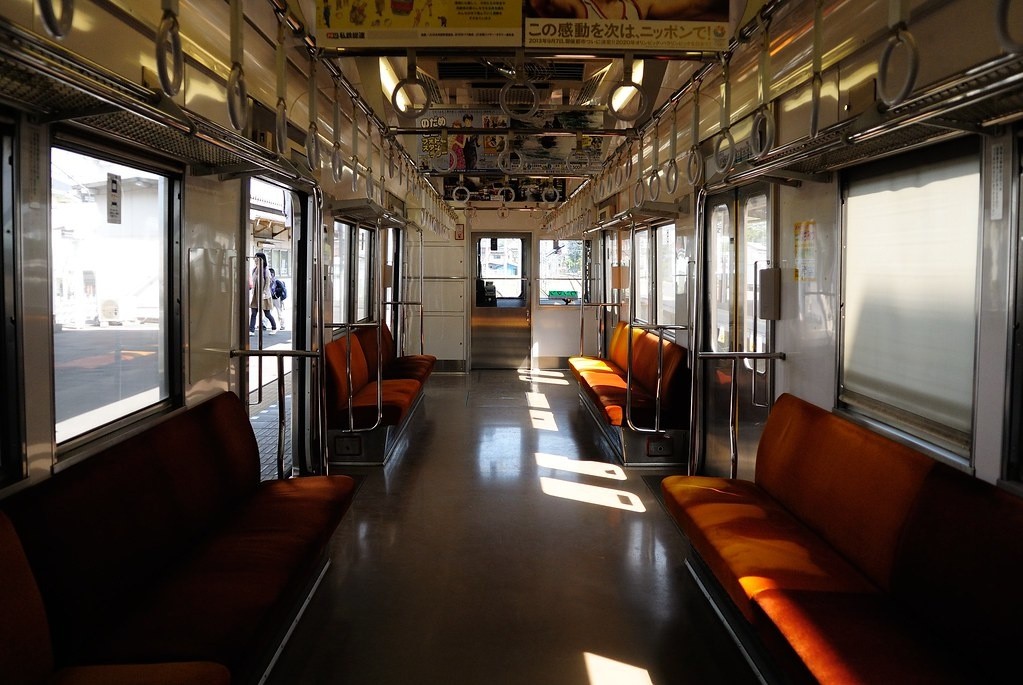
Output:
[275,278,287,301]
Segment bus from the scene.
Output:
[482,258,519,285]
[629,237,769,373]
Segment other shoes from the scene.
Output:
[249,332,255,336]
[280,326,285,330]
[263,326,266,330]
[269,328,278,335]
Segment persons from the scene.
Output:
[248,253,286,332]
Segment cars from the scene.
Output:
[540,276,583,299]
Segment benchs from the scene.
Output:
[1,391,357,685]
[567,321,687,426]
[327,319,437,424]
[661,393,1023,685]
